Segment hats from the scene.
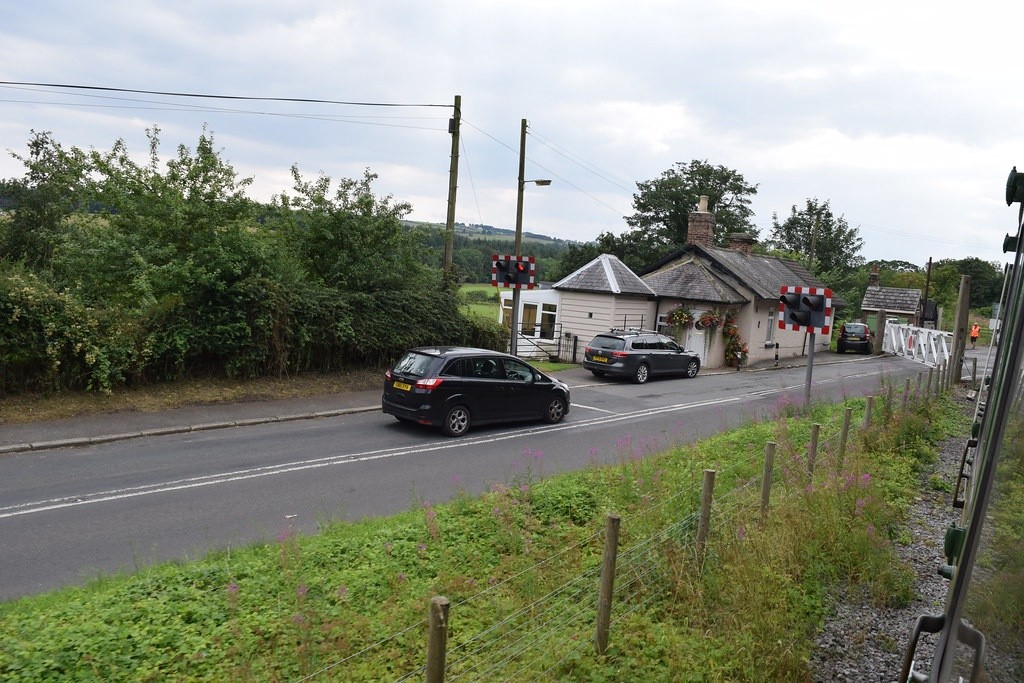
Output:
[974,323,978,326]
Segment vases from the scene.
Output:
[731,356,738,368]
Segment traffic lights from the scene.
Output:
[491,255,535,290]
[776,284,831,335]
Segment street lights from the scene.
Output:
[508,178,551,366]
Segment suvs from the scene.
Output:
[836,321,876,355]
[581,326,702,383]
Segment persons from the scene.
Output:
[970,323,980,349]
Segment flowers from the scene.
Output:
[723,312,738,338]
[698,309,722,330]
[725,334,750,369]
[664,306,694,329]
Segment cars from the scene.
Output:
[380,345,571,437]
